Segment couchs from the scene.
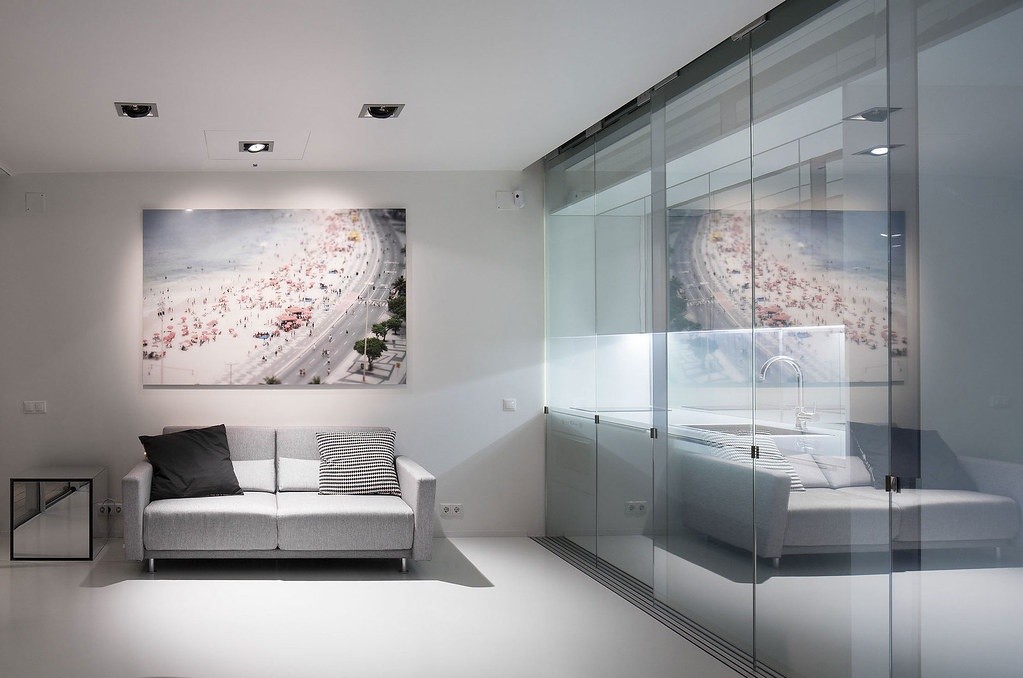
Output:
[682,432,1023,569]
[123,425,438,575]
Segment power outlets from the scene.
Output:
[636,501,648,512]
[440,503,452,517]
[625,501,636,513]
[453,503,465,517]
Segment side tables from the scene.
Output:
[9,465,109,560]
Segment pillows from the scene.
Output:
[316,430,402,497]
[846,421,979,492]
[138,424,244,502]
[700,427,807,492]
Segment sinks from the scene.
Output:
[670,420,847,456]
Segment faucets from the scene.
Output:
[759,355,816,431]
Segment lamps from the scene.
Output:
[358,104,405,119]
[114,102,158,118]
[239,141,274,153]
